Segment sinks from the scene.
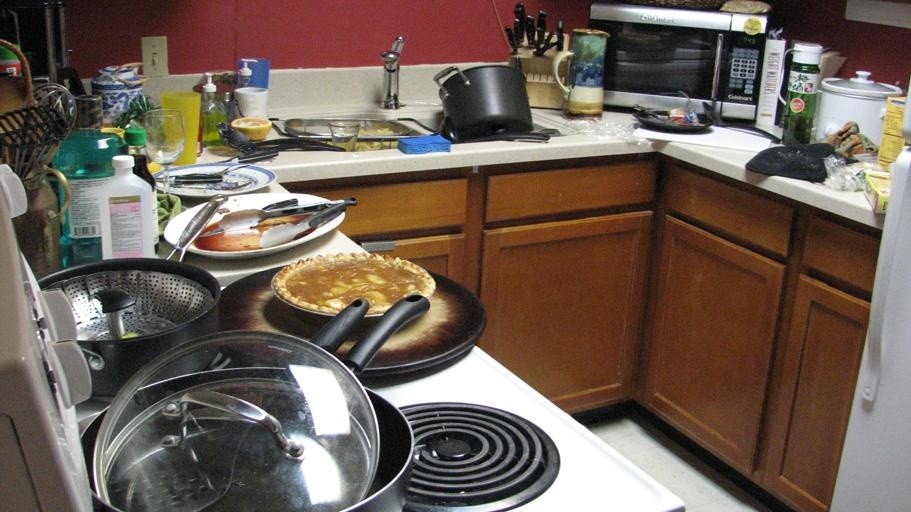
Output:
[272,109,580,153]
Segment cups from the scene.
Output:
[160,91,201,166]
[235,87,269,116]
[240,58,269,89]
[145,109,186,194]
[328,120,360,151]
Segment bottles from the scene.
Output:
[54,96,123,269]
[781,43,823,147]
[124,128,159,253]
[100,154,155,260]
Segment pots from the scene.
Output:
[79,292,430,511]
[433,56,534,137]
[27,193,228,398]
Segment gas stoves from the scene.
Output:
[393,402,561,512]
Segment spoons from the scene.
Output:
[215,122,347,152]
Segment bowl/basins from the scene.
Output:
[231,117,272,139]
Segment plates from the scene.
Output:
[270,257,437,319]
[630,109,712,132]
[163,192,346,261]
[154,162,277,199]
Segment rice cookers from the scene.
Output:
[816,70,904,156]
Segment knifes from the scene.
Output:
[200,199,299,236]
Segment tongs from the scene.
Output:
[218,196,357,250]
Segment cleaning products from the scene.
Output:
[200,71,229,147]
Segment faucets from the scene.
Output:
[379,37,405,109]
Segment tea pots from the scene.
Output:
[552,28,610,120]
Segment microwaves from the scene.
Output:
[587,2,767,124]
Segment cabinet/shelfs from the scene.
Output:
[477,166,656,421]
[293,183,473,299]
[638,150,883,512]
